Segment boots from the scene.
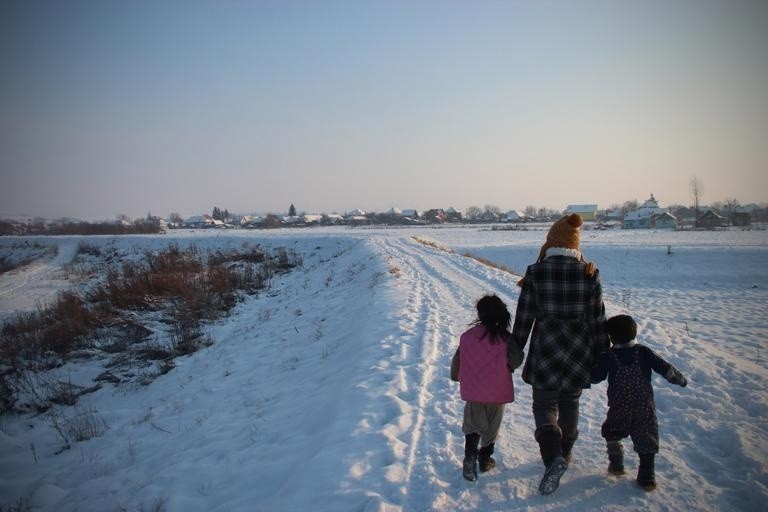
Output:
[556,425,578,466]
[635,451,658,491]
[479,443,497,473]
[606,441,626,476]
[534,423,568,496]
[461,433,478,483]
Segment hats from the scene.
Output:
[546,214,582,252]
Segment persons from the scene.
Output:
[601,314,687,491]
[513,213,612,496]
[450,292,525,482]
